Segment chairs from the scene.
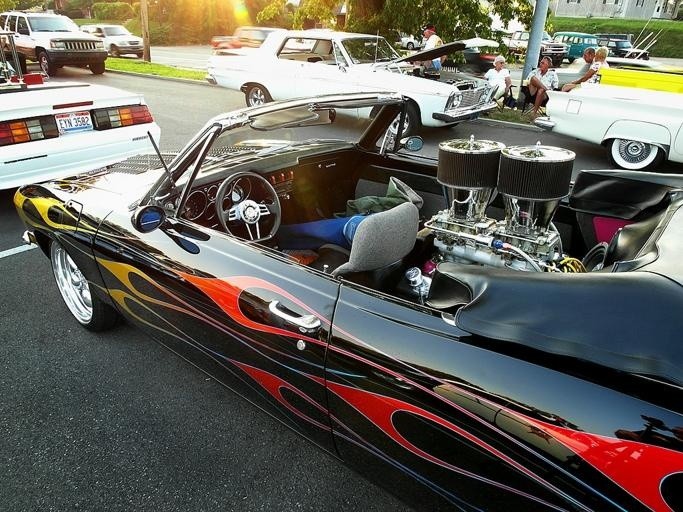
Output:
[493,69,518,114]
[303,200,421,278]
[520,65,560,117]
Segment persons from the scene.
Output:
[583,48,610,70]
[561,46,609,92]
[483,55,512,102]
[420,25,447,70]
[525,55,559,117]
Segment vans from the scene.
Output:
[79,23,144,60]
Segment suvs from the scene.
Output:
[0,11,111,78]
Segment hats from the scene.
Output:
[424,24,438,35]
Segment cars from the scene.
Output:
[494,28,651,65]
[208,26,289,53]
[0,31,163,193]
[206,30,499,138]
[539,83,683,170]
[591,63,683,94]
[350,345,593,477]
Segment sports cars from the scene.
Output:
[6,94,683,512]
